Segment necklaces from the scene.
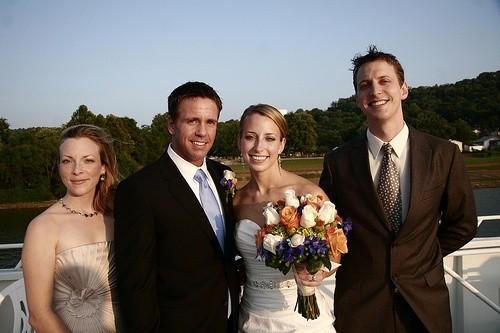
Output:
[59,197,102,217]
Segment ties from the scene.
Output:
[194,168,225,253]
[375,142,403,235]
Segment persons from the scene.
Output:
[230,103,345,333]
[319,45,478,333]
[113,82,248,333]
[21,124,128,333]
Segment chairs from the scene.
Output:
[0,277,38,333]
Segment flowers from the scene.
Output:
[220,169,238,205]
[254,188,354,322]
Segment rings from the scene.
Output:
[312,274,317,281]
